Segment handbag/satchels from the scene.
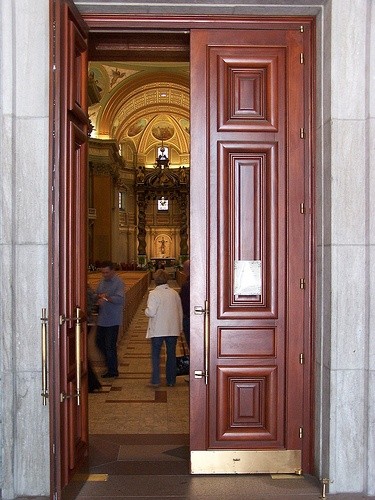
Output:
[176,355,190,376]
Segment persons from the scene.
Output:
[141,269,183,387]
[95,261,125,379]
[86,282,102,392]
[179,259,190,345]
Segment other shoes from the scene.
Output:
[101,371,118,377]
[88,380,102,393]
[167,383,174,387]
[147,381,159,387]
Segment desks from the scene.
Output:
[150,257,176,272]
